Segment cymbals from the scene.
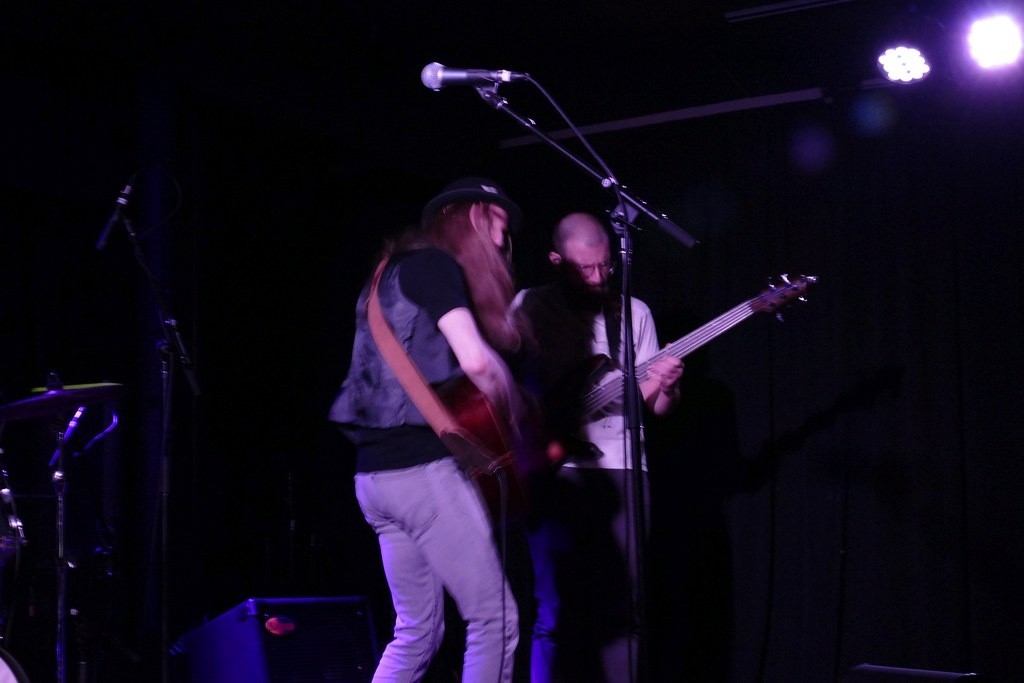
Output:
[0,381,123,422]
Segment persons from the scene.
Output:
[330,177,522,683]
[505,212,685,683]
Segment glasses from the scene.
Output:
[562,259,616,278]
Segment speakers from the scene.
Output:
[186,596,379,683]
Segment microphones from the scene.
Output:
[50,406,86,469]
[421,62,529,91]
[97,185,133,251]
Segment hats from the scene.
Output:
[419,177,522,234]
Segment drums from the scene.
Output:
[0,647,31,683]
[0,452,26,646]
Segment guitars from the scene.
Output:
[502,269,821,537]
[442,373,531,518]
